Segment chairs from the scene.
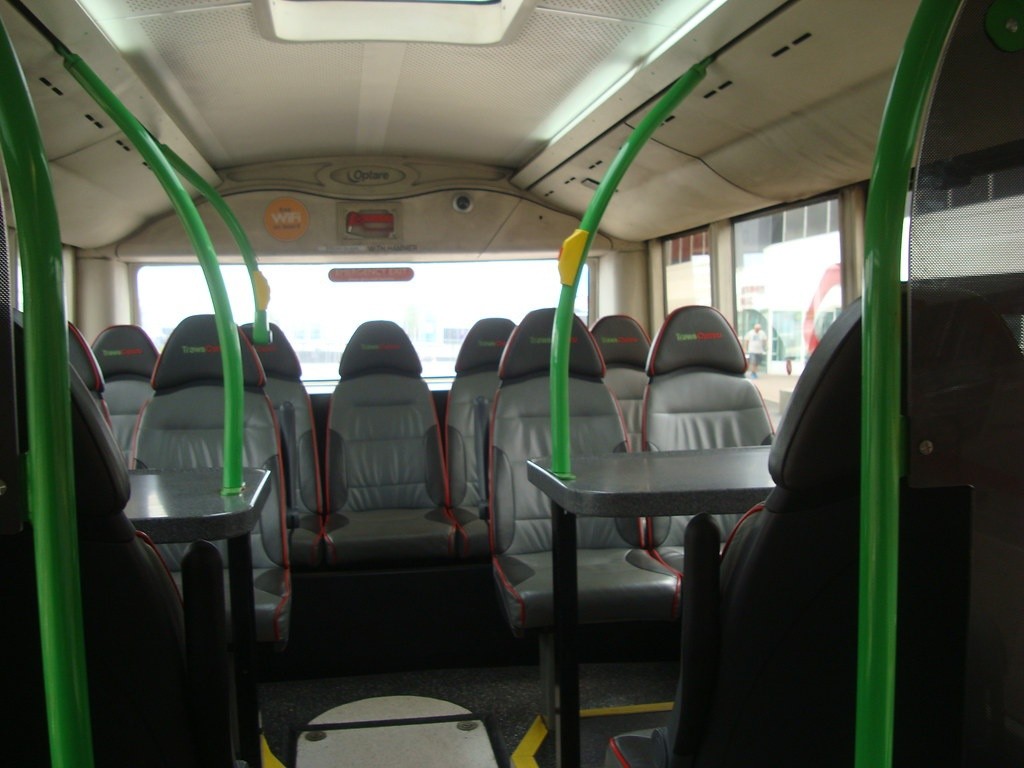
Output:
[0,303,1024,768]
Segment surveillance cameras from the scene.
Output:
[453,193,473,212]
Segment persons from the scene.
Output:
[743,323,768,379]
